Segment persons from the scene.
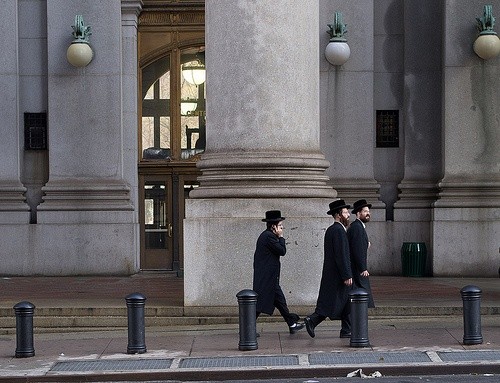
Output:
[252,210,305,338]
[347,199,376,308]
[304,199,352,338]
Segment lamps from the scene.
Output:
[182,54,205,86]
[180,79,197,116]
[472,4,500,59]
[324,11,351,66]
[67,16,94,67]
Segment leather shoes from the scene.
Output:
[289,322,305,334]
[340,329,351,338]
[305,316,316,338]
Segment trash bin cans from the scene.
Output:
[400,241,427,277]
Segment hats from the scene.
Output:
[327,198,351,216]
[261,209,285,222]
[351,198,372,214]
